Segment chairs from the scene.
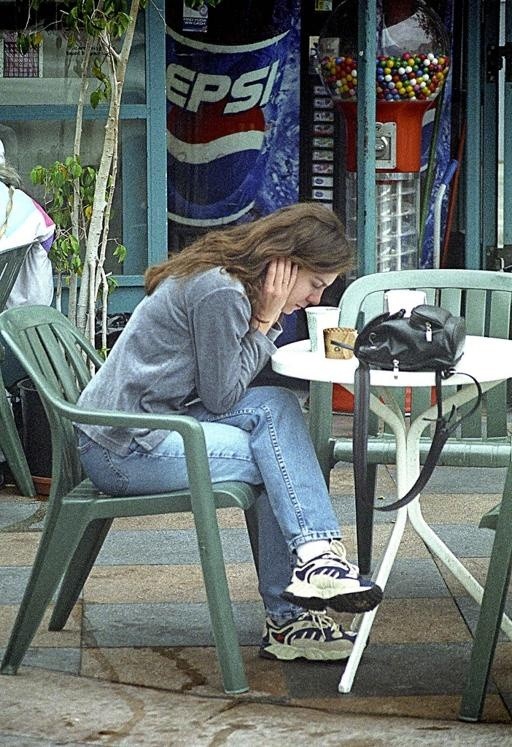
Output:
[306,270,512,500]
[0,243,45,499]
[2,305,266,697]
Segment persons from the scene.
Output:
[0,140,54,389]
[75,203,380,660]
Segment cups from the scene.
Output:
[305,306,340,354]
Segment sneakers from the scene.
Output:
[259,609,369,661]
[280,551,383,613]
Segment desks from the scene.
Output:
[272,324,512,695]
